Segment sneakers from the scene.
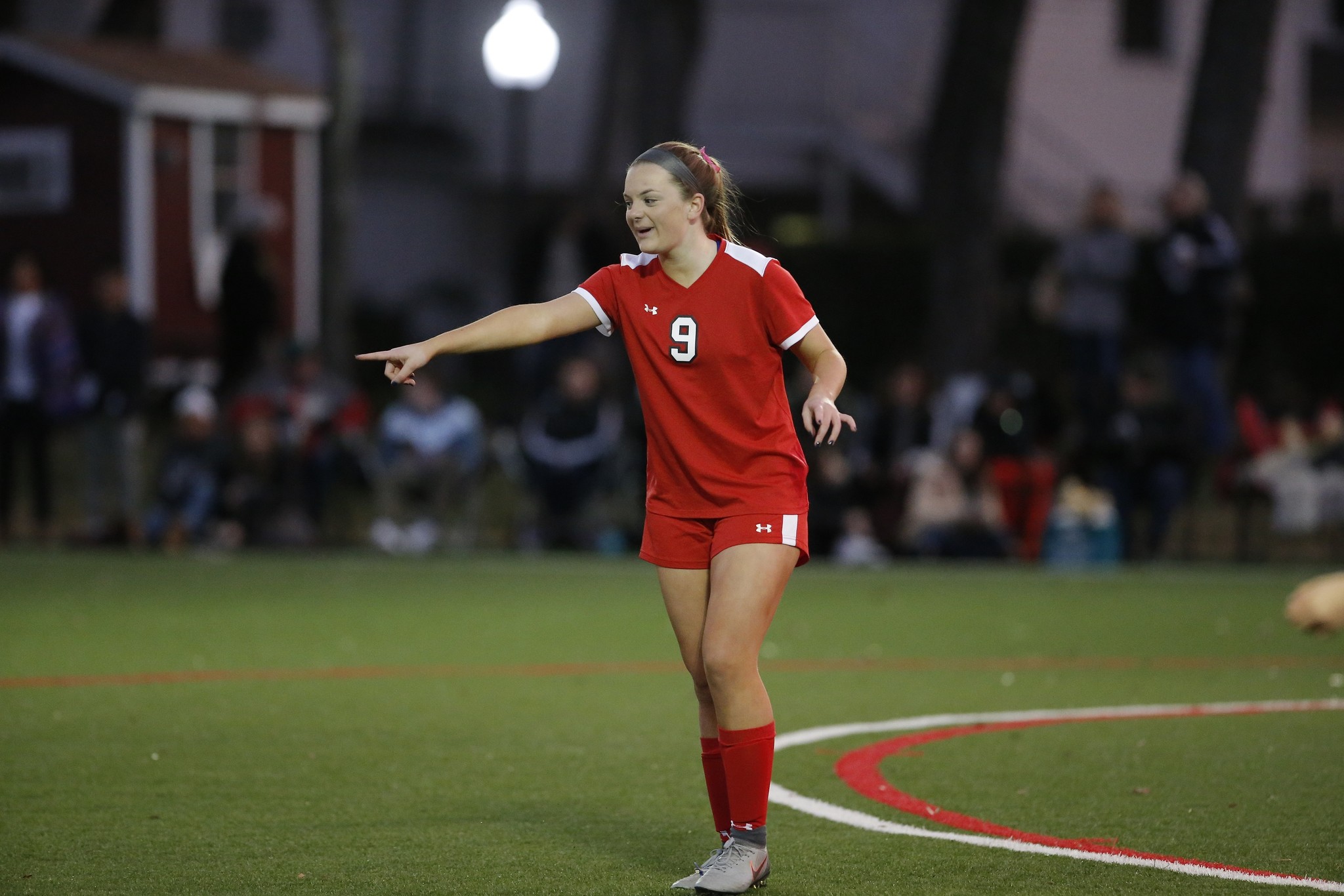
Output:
[670,848,724,890]
[694,843,771,894]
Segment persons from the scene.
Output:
[0,117,1344,572]
[349,140,859,896]
